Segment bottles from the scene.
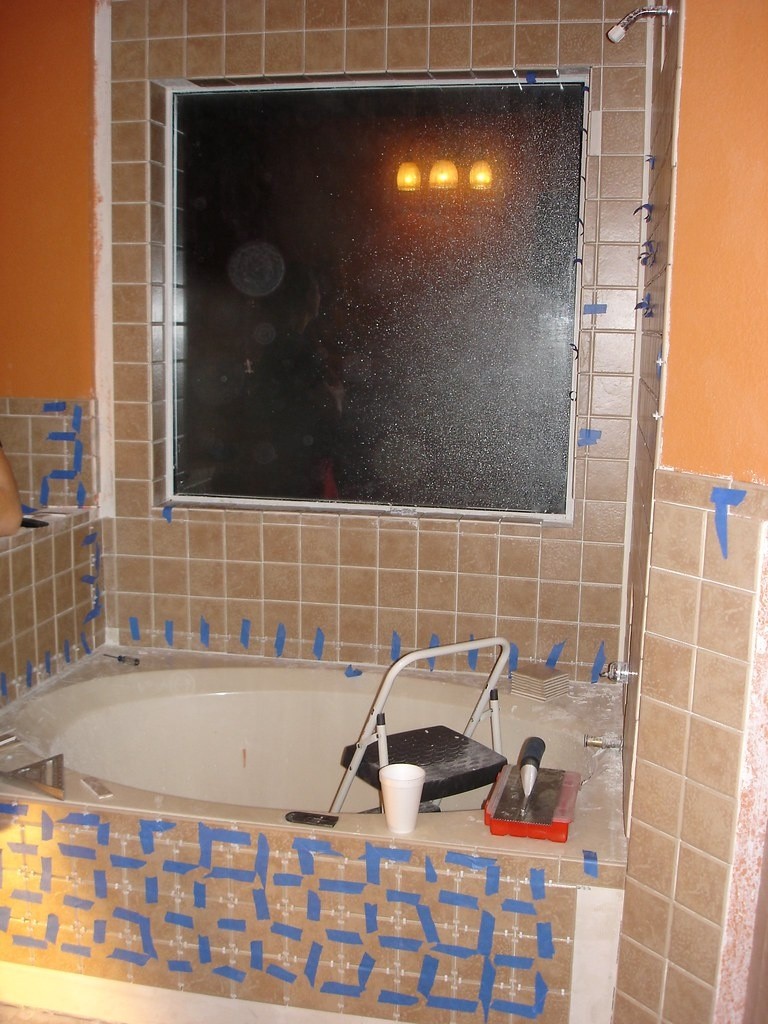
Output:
[0,441,23,537]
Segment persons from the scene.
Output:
[0,442,23,538]
[207,264,348,501]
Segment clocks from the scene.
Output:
[228,239,286,299]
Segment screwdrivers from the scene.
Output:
[102,653,141,667]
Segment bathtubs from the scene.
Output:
[0,646,625,1024]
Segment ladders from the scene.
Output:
[328,635,518,818]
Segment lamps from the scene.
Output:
[429,155,458,191]
[468,155,493,189]
[396,152,422,192]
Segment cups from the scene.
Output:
[380,763,425,834]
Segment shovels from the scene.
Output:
[9,773,65,802]
